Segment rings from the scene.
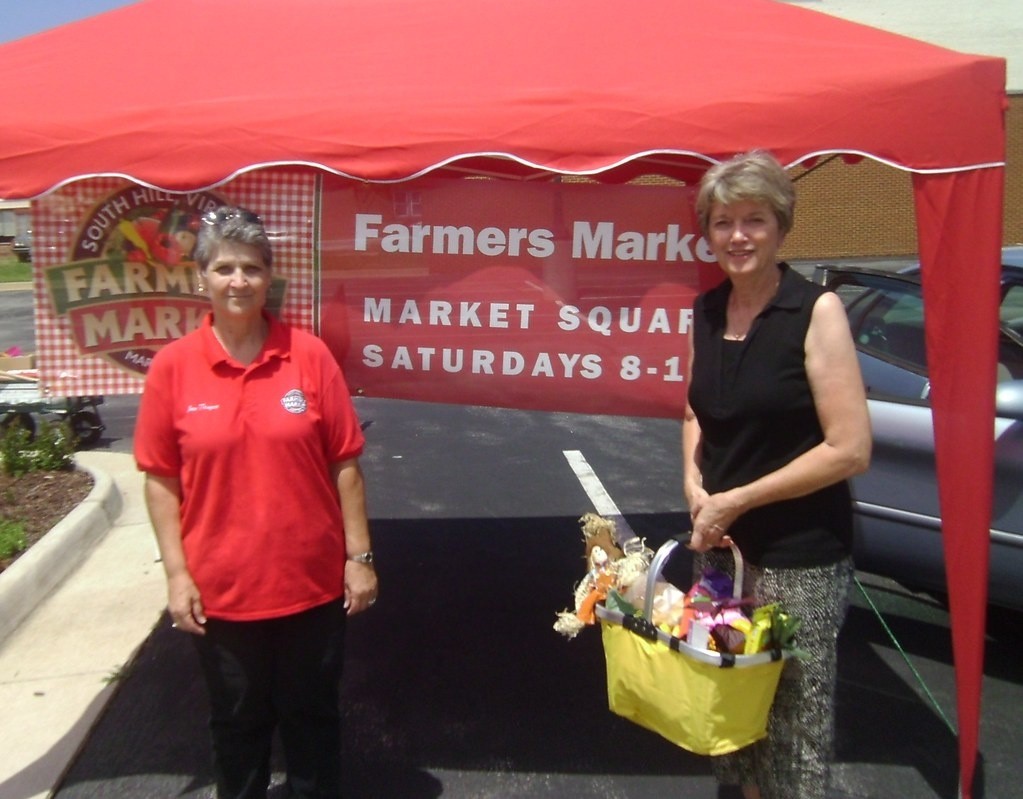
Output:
[368,599,376,606]
[172,623,177,628]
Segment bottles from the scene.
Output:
[679,578,714,641]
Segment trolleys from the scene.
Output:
[0,350,106,452]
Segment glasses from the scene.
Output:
[200,211,263,225]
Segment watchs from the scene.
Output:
[347,553,373,563]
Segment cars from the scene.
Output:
[814,245,1023,641]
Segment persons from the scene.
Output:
[135,206,379,799]
[683,147,873,799]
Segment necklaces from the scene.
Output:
[733,330,744,340]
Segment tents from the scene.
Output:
[0,1,1007,799]
